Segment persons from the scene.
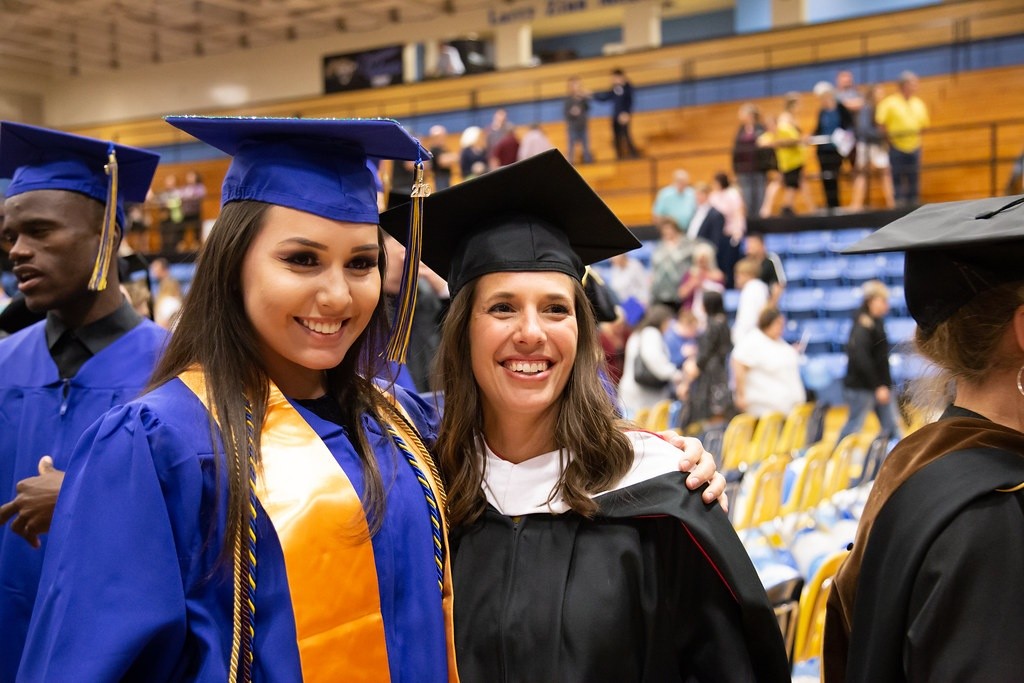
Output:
[597,168,807,431]
[836,279,899,439]
[15,114,729,683]
[732,69,932,218]
[431,109,550,191]
[372,222,451,394]
[378,147,792,683]
[591,68,636,159]
[821,193,1024,683]
[436,39,465,76]
[159,169,207,251]
[0,119,174,683]
[563,75,592,163]
[149,257,182,327]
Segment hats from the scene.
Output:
[163,115,433,365]
[858,280,893,302]
[840,193,1024,326]
[377,148,644,302]
[0,120,161,292]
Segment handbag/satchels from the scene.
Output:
[635,356,669,390]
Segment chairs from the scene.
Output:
[600,226,943,683]
